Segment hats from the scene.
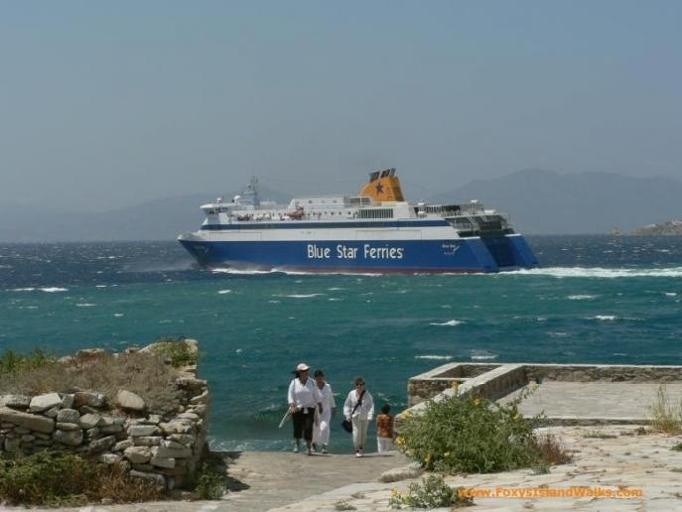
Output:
[292,362,311,373]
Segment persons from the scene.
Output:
[342,376,374,458]
[310,370,337,453]
[375,404,394,454]
[287,362,323,455]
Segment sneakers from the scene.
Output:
[293,445,365,458]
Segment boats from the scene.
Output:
[177,168,541,274]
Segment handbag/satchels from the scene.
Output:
[341,419,353,434]
